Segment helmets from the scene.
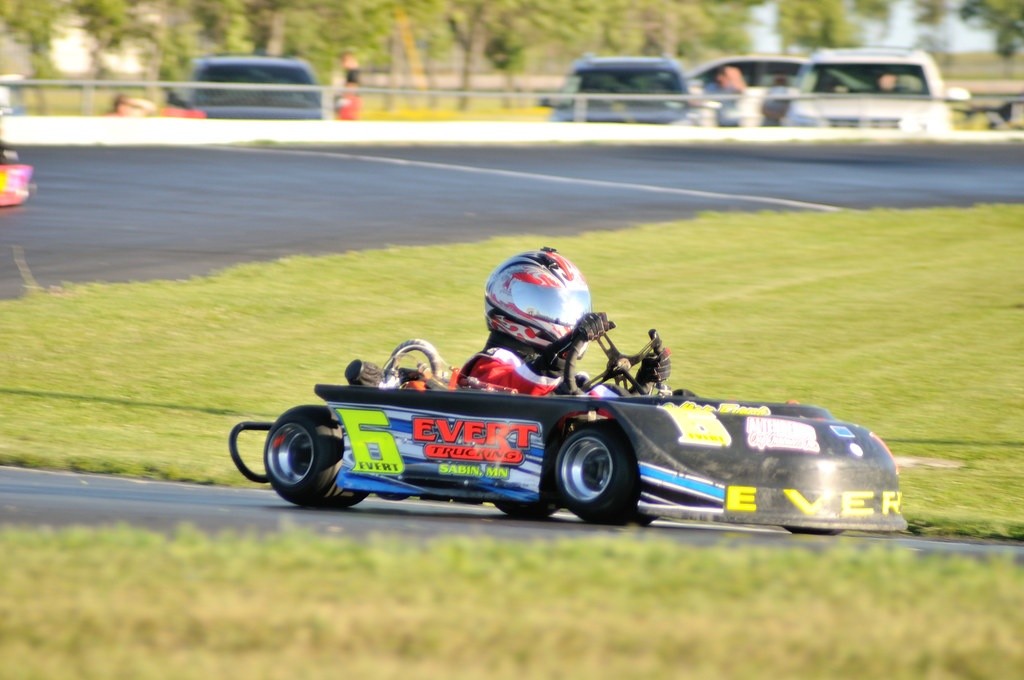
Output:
[484,251,592,360]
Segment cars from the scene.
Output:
[538,50,693,128]
[775,48,972,133]
[166,57,322,121]
[685,52,809,132]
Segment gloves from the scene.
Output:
[640,347,671,383]
[572,312,615,342]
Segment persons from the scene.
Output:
[333,50,361,120]
[456,248,671,397]
